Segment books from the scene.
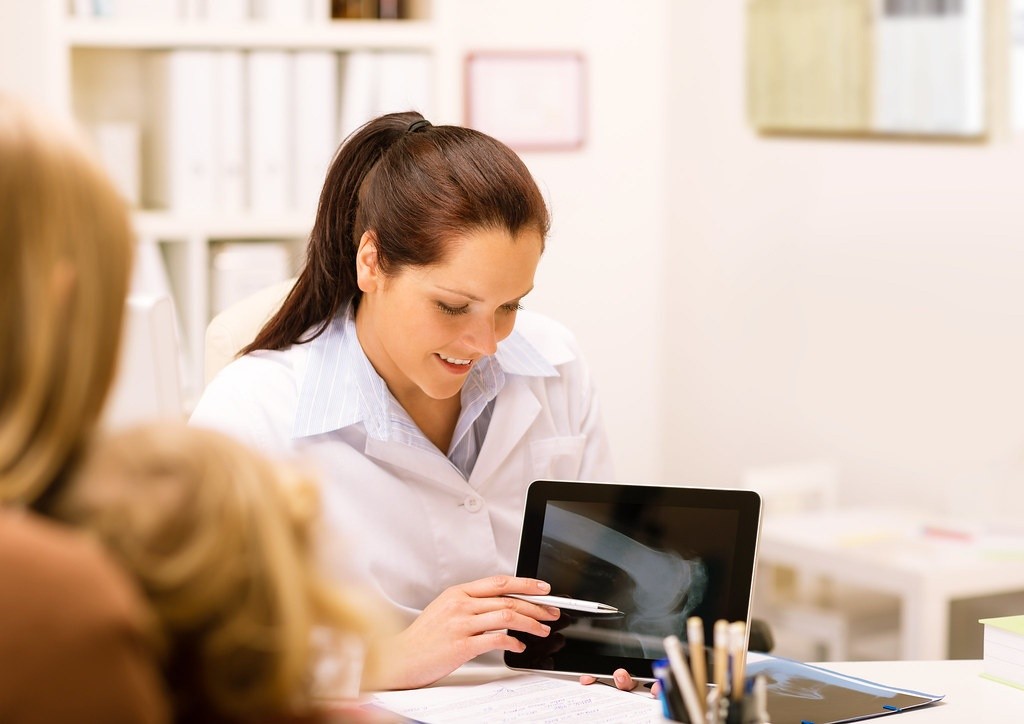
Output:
[978,615,1024,691]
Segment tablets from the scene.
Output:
[502,481,763,688]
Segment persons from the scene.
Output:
[188,112,661,699]
[0,100,384,724]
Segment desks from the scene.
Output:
[364,657,1024,724]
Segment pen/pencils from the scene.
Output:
[651,616,770,724]
[502,592,626,616]
